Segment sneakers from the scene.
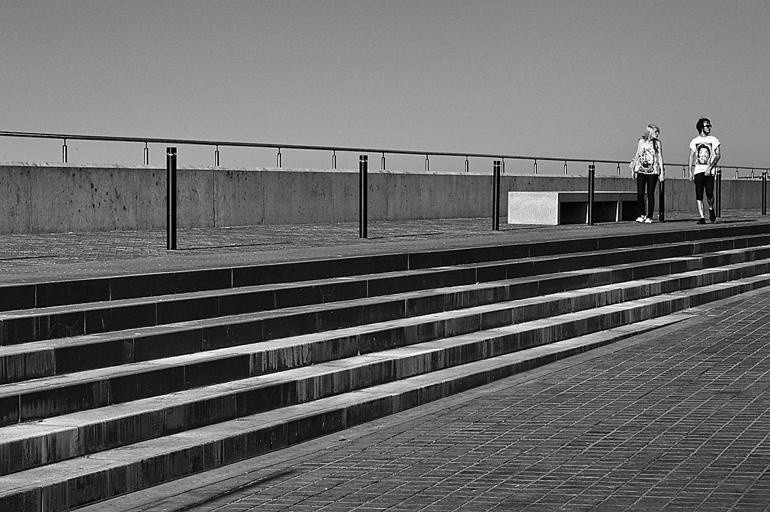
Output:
[636,215,652,224]
[696,209,716,224]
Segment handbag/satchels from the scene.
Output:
[629,159,638,180]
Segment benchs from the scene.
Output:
[507,191,652,225]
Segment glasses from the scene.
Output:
[652,130,660,133]
[703,125,712,128]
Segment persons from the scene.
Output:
[687,117,721,226]
[628,123,665,224]
